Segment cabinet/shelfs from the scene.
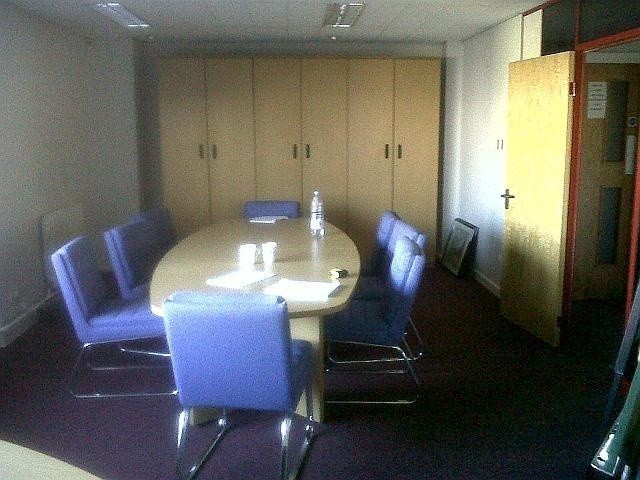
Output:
[253,55,346,236]
[155,56,254,244]
[347,58,442,274]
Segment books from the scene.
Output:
[262,215,289,221]
[249,215,276,225]
[261,277,341,300]
[205,269,277,290]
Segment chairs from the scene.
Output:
[243,200,299,220]
[163,291,316,479]
[353,220,426,360]
[323,235,427,405]
[105,219,150,300]
[134,207,179,262]
[49,235,179,397]
[359,209,401,278]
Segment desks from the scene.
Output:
[151,217,360,425]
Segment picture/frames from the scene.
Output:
[440,217,479,276]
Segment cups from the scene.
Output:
[237,245,260,264]
[262,240,277,266]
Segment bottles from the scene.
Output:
[310,191,324,237]
